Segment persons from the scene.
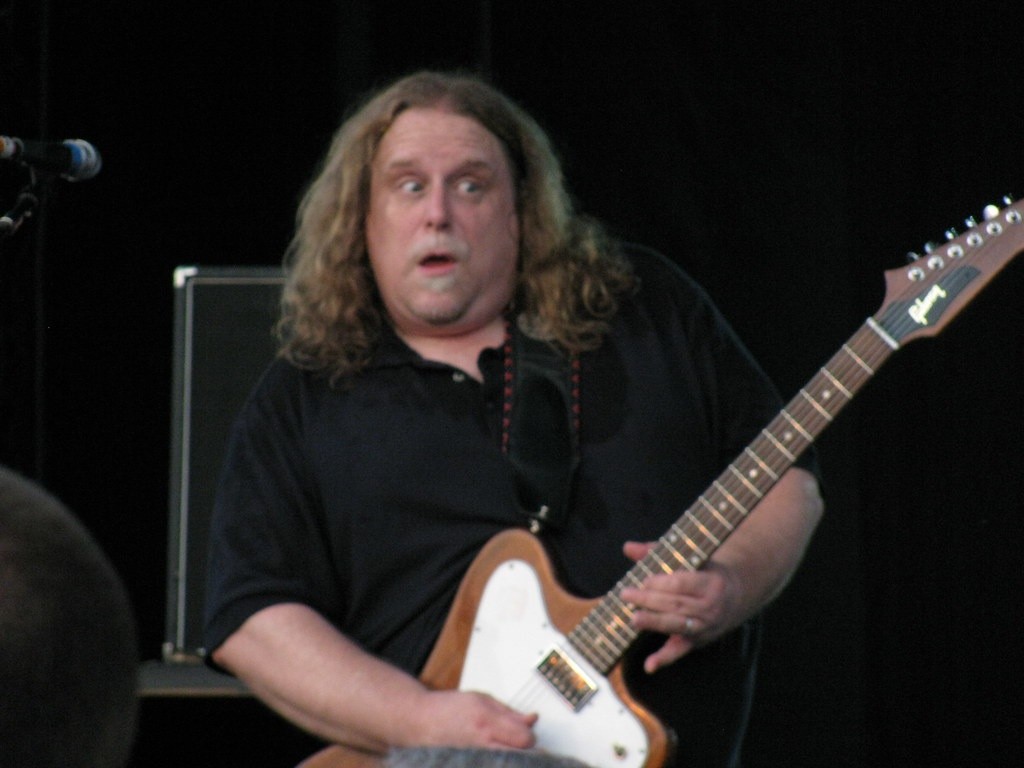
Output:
[198,71,827,757]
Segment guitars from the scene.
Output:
[295,186,1022,765]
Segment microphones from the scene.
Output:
[0,134,101,183]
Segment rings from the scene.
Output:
[683,617,693,637]
